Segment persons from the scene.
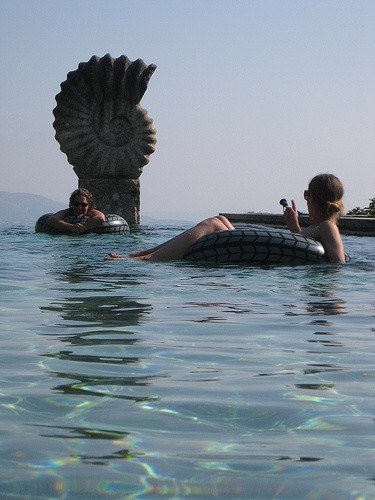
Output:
[104,174,346,266]
[46,188,106,236]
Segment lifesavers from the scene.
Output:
[182,228,352,267]
[35,212,130,234]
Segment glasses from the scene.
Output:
[304,190,312,201]
[71,202,90,207]
